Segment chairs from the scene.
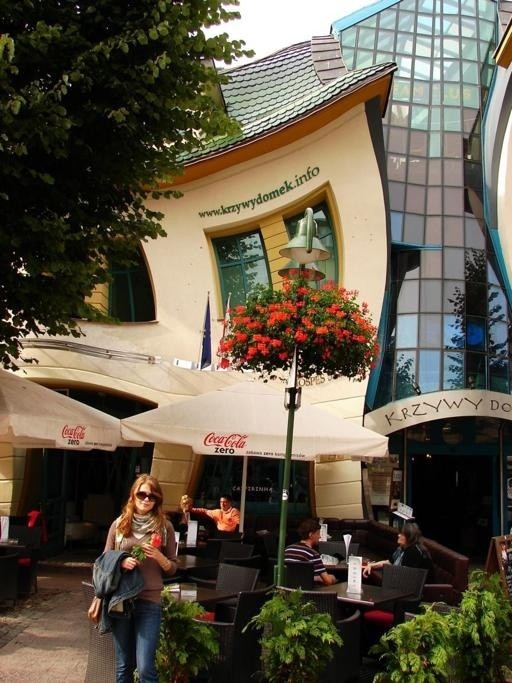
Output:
[81,533,428,683]
[0,516,41,608]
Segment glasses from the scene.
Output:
[136,491,158,502]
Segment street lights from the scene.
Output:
[276,202,332,592]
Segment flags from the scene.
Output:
[219,299,232,369]
[201,301,211,369]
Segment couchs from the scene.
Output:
[312,518,469,607]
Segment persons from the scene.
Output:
[283,520,336,586]
[167,494,240,535]
[362,522,439,613]
[103,474,177,683]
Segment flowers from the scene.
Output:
[216,270,380,383]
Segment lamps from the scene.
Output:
[411,380,421,395]
[467,377,475,389]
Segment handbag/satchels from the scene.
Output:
[88,596,101,625]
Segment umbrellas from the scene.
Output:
[0,369,144,452]
[121,381,389,587]
